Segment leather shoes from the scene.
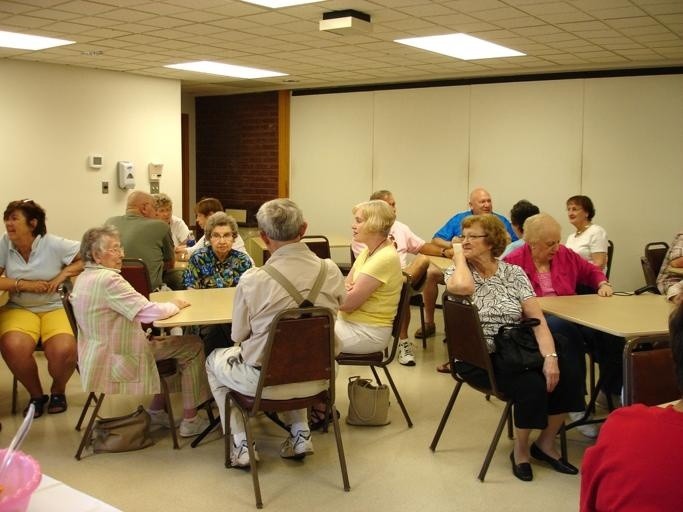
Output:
[532,441,579,475]
[511,447,533,481]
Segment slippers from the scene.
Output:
[24,393,68,417]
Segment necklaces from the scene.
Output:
[576,223,591,237]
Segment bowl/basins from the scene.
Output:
[0,448,43,512]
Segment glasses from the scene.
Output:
[390,234,398,250]
[460,234,486,241]
[22,198,35,207]
[108,247,125,254]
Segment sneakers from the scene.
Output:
[228,439,260,466]
[146,408,180,429]
[180,416,219,437]
[280,428,314,459]
[571,408,598,438]
[595,390,622,410]
[415,322,436,337]
[398,337,416,365]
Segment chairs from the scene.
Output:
[350,245,427,349]
[336,272,412,429]
[430,292,568,481]
[606,240,614,280]
[1,258,179,460]
[223,306,350,509]
[300,236,331,261]
[641,242,670,293]
[624,333,677,407]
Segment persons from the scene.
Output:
[308,201,403,431]
[69,224,212,437]
[579,300,683,511]
[655,233,683,303]
[498,199,539,261]
[0,198,86,419]
[565,195,608,294]
[502,212,624,438]
[413,189,518,339]
[103,191,253,349]
[350,189,453,369]
[444,213,585,480]
[204,198,349,466]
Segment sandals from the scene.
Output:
[437,361,457,373]
[308,404,341,428]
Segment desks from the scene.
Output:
[428,255,454,276]
[248,237,351,276]
[28,473,124,511]
[538,291,679,433]
[149,286,293,448]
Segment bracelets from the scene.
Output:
[15,278,23,293]
[599,282,612,288]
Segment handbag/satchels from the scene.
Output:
[347,376,390,426]
[91,405,154,452]
[493,319,570,372]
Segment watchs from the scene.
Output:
[544,352,558,359]
